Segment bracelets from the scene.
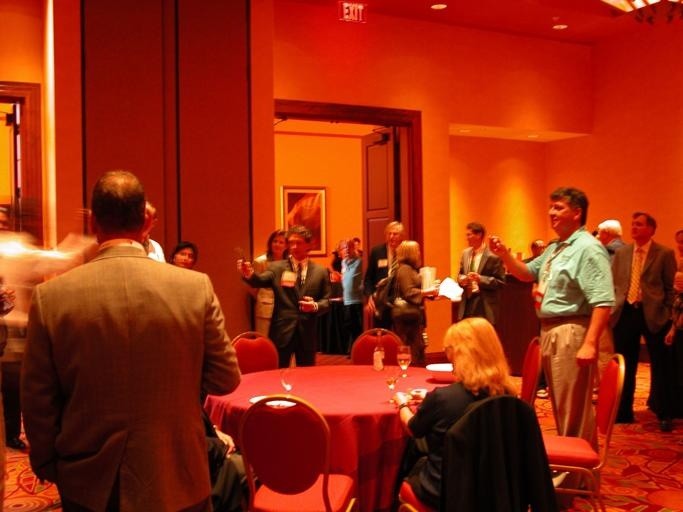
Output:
[398,403,408,409]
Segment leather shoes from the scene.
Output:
[5,435,26,450]
[658,417,673,435]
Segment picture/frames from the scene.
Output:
[280,184,327,258]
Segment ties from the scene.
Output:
[389,246,399,277]
[469,252,476,276]
[295,265,305,289]
[627,250,643,304]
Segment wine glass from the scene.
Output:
[397,346,412,378]
[279,369,295,394]
[384,376,397,403]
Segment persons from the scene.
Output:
[19,169,240,512]
[198,401,270,505]
[455,222,506,329]
[392,317,521,512]
[606,210,676,433]
[364,222,439,369]
[169,239,199,269]
[140,198,165,264]
[327,237,365,354]
[237,225,332,368]
[0,360,29,450]
[665,229,682,346]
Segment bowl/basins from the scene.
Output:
[425,364,454,381]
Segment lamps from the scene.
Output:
[600,0,682,13]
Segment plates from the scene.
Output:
[249,396,296,409]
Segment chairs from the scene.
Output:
[350,328,403,366]
[239,393,356,512]
[231,330,279,375]
[542,353,626,512]
[520,337,542,407]
